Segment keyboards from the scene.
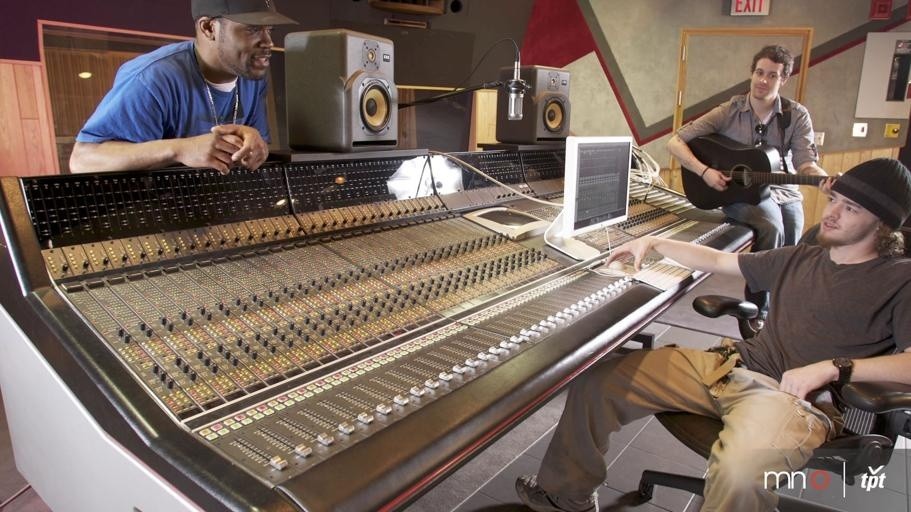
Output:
[632,256,697,293]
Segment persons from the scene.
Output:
[516,157,911,512]
[666,45,838,339]
[69,0,299,174]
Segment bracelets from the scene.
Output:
[701,167,710,177]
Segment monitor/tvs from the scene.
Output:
[543,135,633,262]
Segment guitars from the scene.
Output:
[682,131,841,210]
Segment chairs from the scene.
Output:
[637,220,910,511]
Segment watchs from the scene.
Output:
[833,357,853,383]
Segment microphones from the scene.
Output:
[506,53,529,122]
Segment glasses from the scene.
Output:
[754,119,768,146]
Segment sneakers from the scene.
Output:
[515,475,599,512]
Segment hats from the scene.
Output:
[191,1,300,26]
[830,158,911,232]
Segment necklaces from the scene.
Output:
[191,43,239,127]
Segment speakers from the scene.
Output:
[284,27,400,152]
[495,64,571,145]
